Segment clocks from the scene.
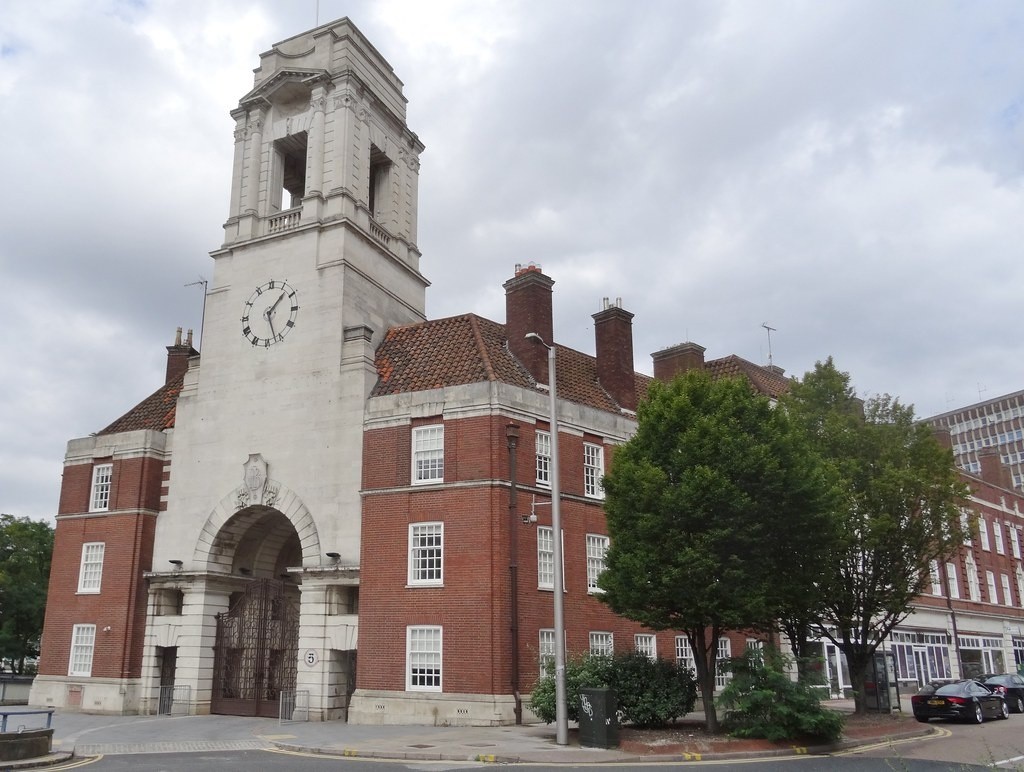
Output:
[239,278,301,349]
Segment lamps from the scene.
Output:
[168,559,183,570]
[103,625,111,632]
[326,552,341,564]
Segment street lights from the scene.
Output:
[525,331,569,746]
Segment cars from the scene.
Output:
[910,679,1009,725]
[974,673,1024,713]
